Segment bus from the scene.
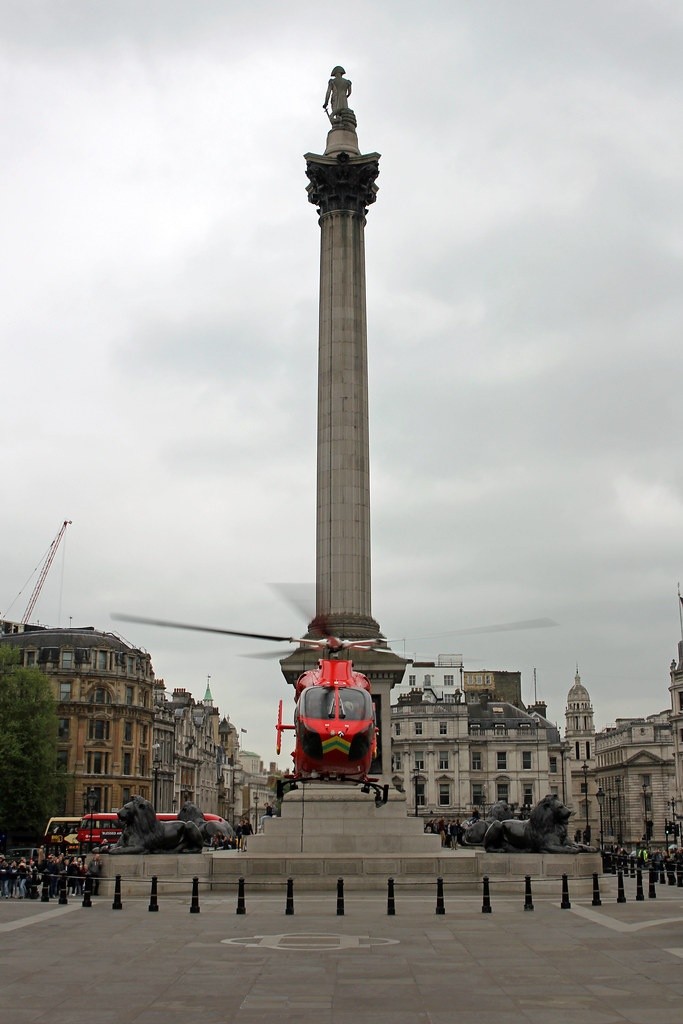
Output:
[43,816,115,857]
[75,812,237,852]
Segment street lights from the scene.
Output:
[641,783,648,850]
[253,793,260,835]
[596,786,606,853]
[413,766,421,817]
[85,786,99,854]
[480,794,487,820]
[581,760,590,846]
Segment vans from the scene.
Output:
[2,848,40,865]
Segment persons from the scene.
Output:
[323,66,352,114]
[423,806,481,850]
[0,826,108,900]
[217,803,273,853]
[605,845,683,861]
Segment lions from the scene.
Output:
[108,794,233,854]
[461,793,598,854]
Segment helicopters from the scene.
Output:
[104,576,561,810]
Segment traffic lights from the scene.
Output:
[671,824,679,836]
[665,825,670,835]
